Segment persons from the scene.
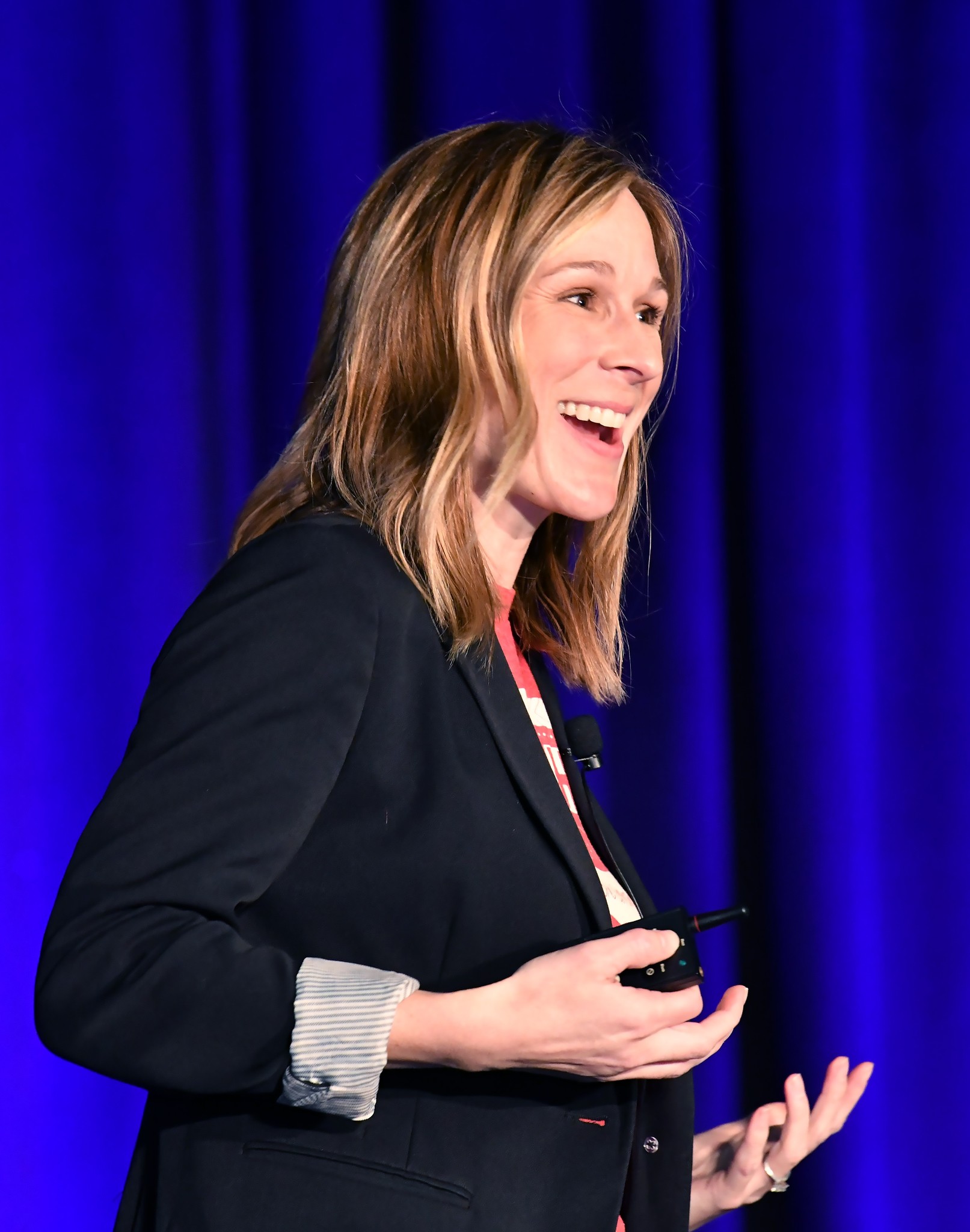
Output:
[30,117,877,1232]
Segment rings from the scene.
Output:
[761,1151,792,1195]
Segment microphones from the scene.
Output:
[565,714,604,769]
